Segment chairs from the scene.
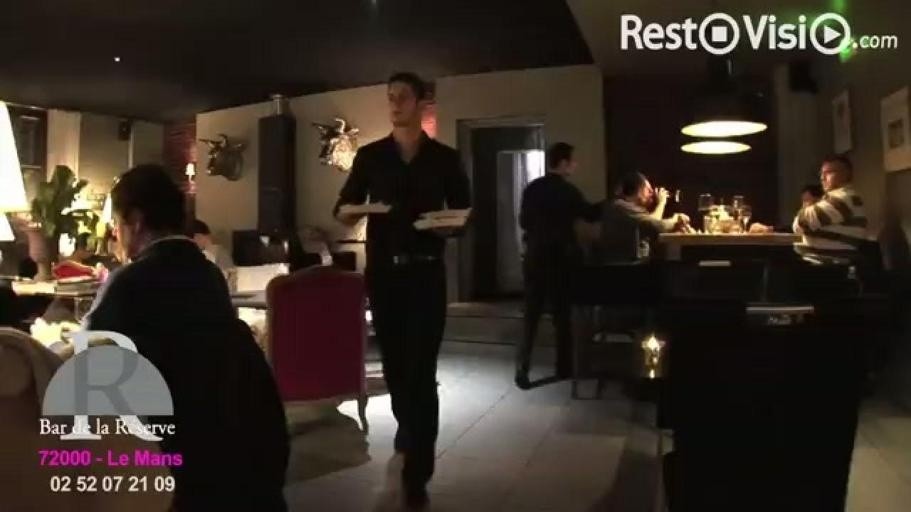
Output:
[567,225,635,402]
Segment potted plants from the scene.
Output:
[7,166,113,283]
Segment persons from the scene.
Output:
[766,155,869,305]
[585,171,692,316]
[47,161,238,427]
[512,141,621,393]
[749,183,826,233]
[0,213,324,367]
[612,173,675,269]
[331,69,472,511]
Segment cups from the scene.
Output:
[732,197,745,216]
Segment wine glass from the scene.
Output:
[738,203,752,233]
[697,194,715,233]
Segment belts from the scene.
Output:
[365,255,442,265]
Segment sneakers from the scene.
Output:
[515,370,530,389]
[400,468,431,512]
[393,425,408,452]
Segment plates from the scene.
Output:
[413,207,473,231]
[337,202,392,217]
